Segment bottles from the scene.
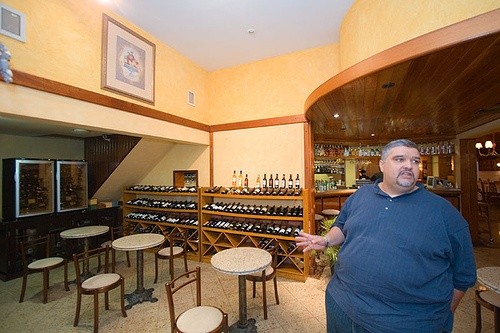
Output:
[202,171,303,254]
[19,169,49,209]
[344,140,454,156]
[60,168,83,210]
[125,183,198,248]
[313,143,345,192]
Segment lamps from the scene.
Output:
[475,129,497,157]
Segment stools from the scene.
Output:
[315,214,323,259]
[321,209,339,231]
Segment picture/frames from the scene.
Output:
[98,13,157,106]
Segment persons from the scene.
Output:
[295,140,477,333]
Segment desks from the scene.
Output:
[110,233,165,310]
[210,247,273,333]
[59,226,110,284]
[476,265,500,294]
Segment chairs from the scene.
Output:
[17,231,132,333]
[153,227,279,333]
[474,289,500,333]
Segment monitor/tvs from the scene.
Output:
[427,176,438,189]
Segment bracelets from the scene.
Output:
[323,236,329,247]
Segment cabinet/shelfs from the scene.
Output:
[0,205,120,283]
[200,186,308,282]
[119,186,201,262]
